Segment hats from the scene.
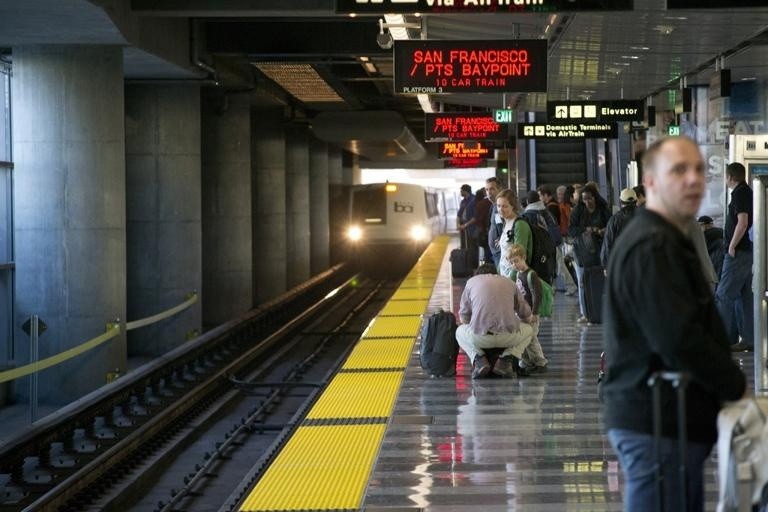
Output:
[461,184,472,192]
[620,188,637,203]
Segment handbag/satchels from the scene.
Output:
[420,310,459,376]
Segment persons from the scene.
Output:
[694,213,728,285]
[711,162,756,354]
[594,133,749,511]
[452,175,647,379]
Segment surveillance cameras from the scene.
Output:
[377,33,393,50]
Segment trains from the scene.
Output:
[348,183,463,265]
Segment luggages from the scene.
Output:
[583,265,605,324]
[451,228,474,278]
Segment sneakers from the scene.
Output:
[518,366,545,375]
[565,286,577,296]
[577,316,588,323]
[493,359,517,377]
[731,338,755,352]
[471,355,491,379]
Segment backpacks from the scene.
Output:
[713,396,768,511]
[526,270,554,318]
[509,215,556,277]
[527,211,562,245]
